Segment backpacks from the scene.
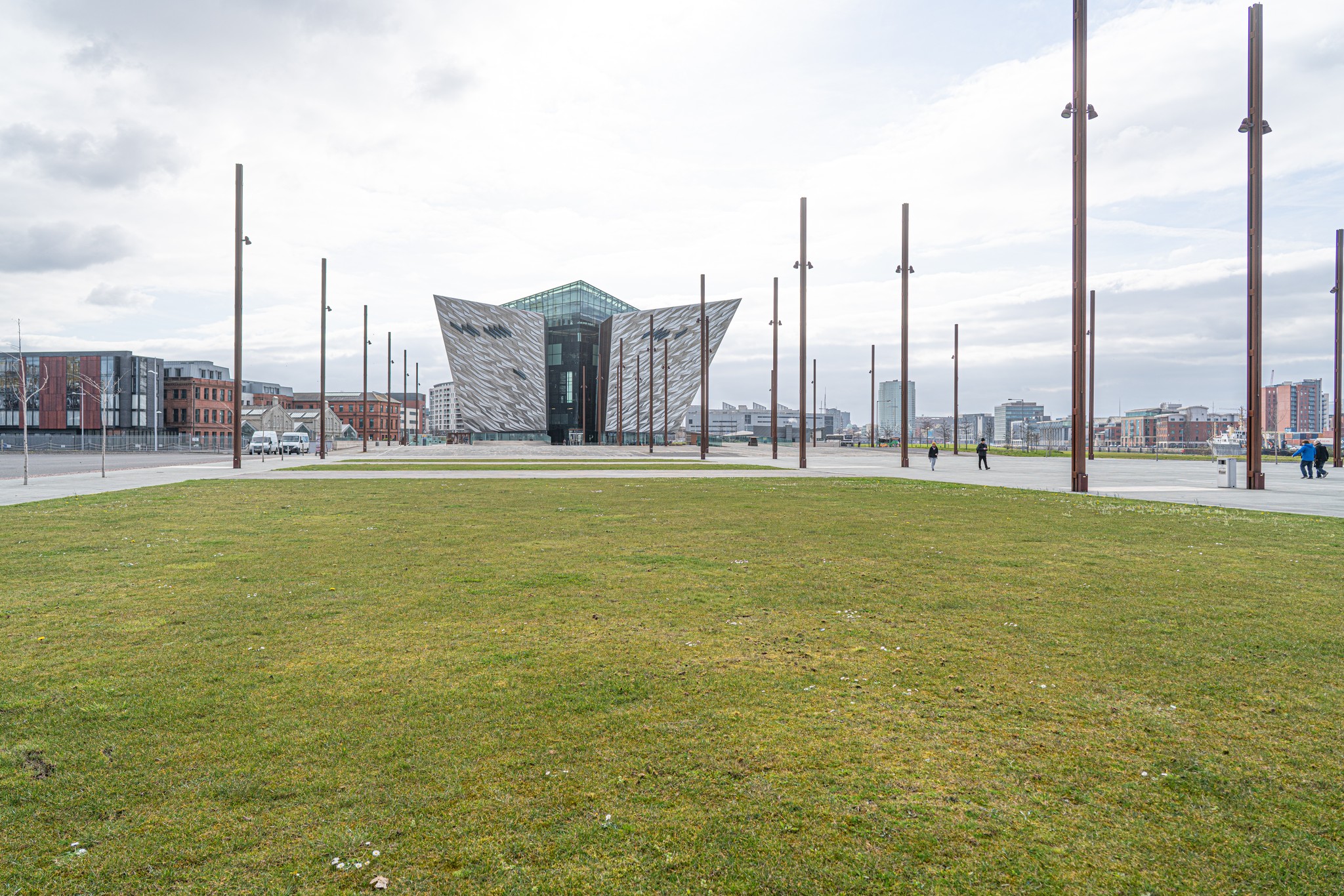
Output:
[976,443,985,453]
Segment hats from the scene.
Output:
[932,442,936,446]
[981,438,985,442]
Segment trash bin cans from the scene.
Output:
[689,442,691,445]
[1217,457,1236,488]
[376,442,379,446]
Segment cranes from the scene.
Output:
[1219,407,1247,414]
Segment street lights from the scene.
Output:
[232,163,252,468]
[1181,429,1184,448]
[71,382,86,451]
[415,363,421,446]
[388,332,394,446]
[430,422,438,436]
[450,413,463,444]
[579,197,891,468]
[320,257,332,459]
[1237,3,1273,489]
[403,349,410,446]
[1008,399,1023,446]
[363,305,371,452]
[952,324,959,454]
[1329,229,1344,468]
[1062,0,1097,492]
[895,201,915,467]
[148,357,162,451]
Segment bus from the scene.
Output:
[824,433,854,446]
[876,436,901,447]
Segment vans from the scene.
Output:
[1289,446,1302,450]
[248,431,278,455]
[279,432,309,455]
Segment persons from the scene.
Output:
[928,442,938,471]
[630,439,632,445]
[564,439,566,446]
[857,441,860,447]
[626,439,628,445]
[402,436,404,445]
[977,438,990,470]
[1314,440,1329,479]
[1292,440,1316,479]
[1293,446,1293,447]
[696,439,699,446]
[392,437,395,441]
[1286,445,1288,447]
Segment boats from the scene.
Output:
[1205,406,1273,455]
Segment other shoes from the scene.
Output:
[979,468,982,470]
[1322,472,1328,478]
[986,467,990,470]
[932,468,934,471]
[1306,477,1313,479]
[1315,476,1322,478]
[1300,476,1308,478]
